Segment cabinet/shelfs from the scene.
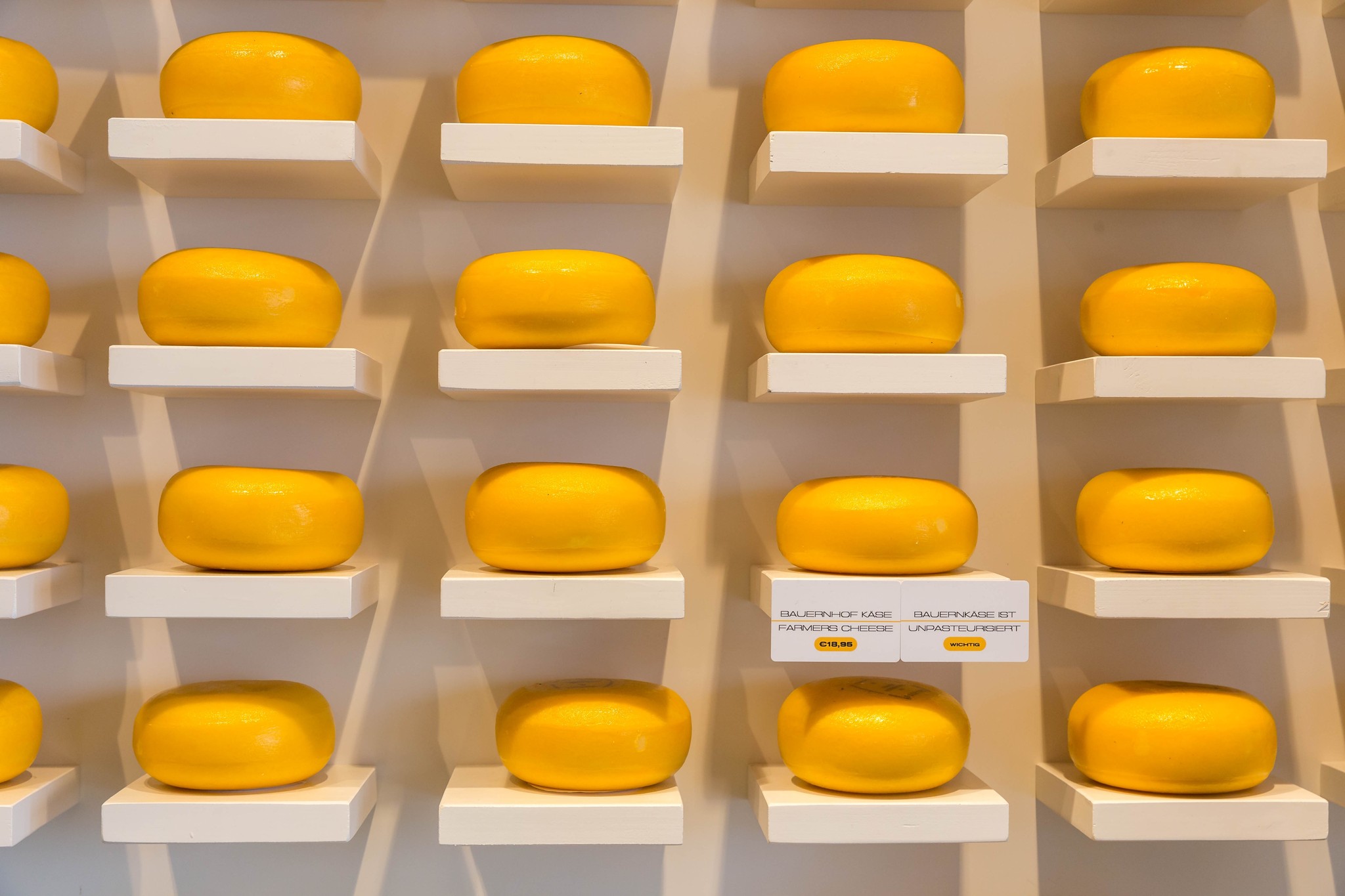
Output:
[0,121,86,848]
[100,119,381,845]
[747,132,1010,846]
[436,124,687,846]
[1037,136,1333,845]
